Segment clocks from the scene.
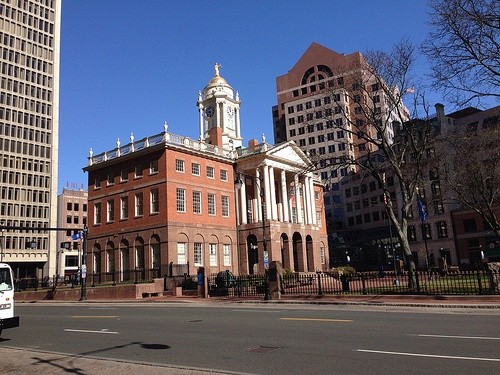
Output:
[204,106,215,119]
[226,105,234,119]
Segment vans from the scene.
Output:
[0,263,14,334]
